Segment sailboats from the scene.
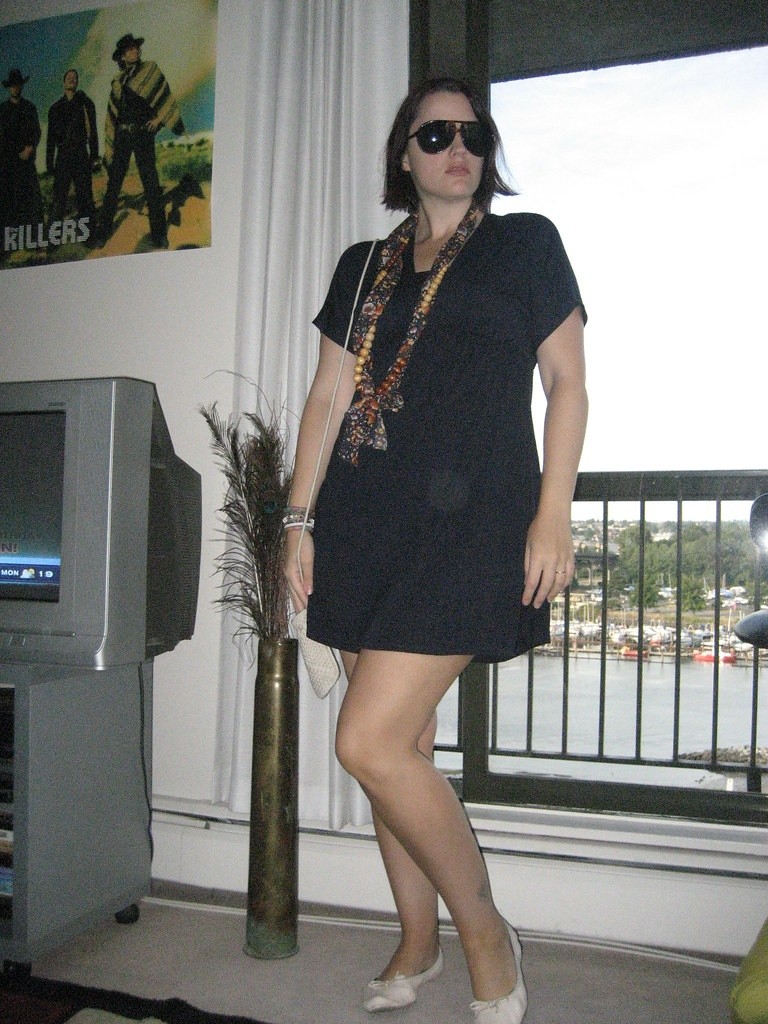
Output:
[528,573,768,667]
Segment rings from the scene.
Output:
[555,571,565,574]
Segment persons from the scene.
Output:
[282,78,589,1024]
[46,70,101,253]
[98,34,184,248]
[0,69,43,229]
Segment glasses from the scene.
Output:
[408,119,495,156]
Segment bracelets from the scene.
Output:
[282,505,313,533]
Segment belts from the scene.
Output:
[120,123,141,134]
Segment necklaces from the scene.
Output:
[334,201,485,465]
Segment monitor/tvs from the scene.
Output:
[0,376,202,670]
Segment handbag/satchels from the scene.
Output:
[88,154,102,173]
[291,608,341,700]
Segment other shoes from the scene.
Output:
[154,234,168,248]
[364,945,444,1013]
[469,918,528,1024]
[97,226,113,240]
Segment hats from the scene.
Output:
[2,69,30,86]
[112,34,144,61]
